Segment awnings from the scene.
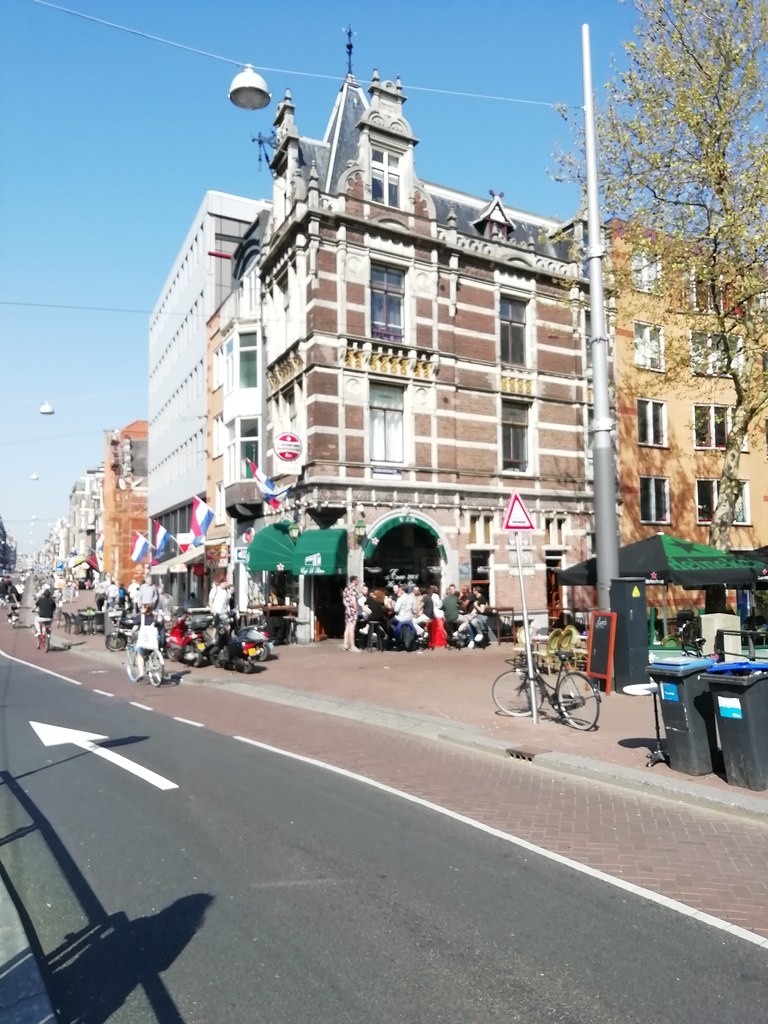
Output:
[246,524,301,571]
[293,529,347,575]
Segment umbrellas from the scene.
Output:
[558,529,768,637]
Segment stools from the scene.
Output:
[367,621,383,654]
[445,621,461,650]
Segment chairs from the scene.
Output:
[512,625,587,673]
[62,612,94,635]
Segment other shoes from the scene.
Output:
[344,645,361,652]
[34,632,41,638]
[468,641,474,648]
[475,634,483,642]
[137,675,144,682]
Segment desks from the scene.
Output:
[485,607,516,645]
[79,610,115,635]
[245,605,298,627]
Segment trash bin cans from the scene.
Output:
[699,661,768,791]
[645,656,718,775]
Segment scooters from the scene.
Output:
[223,613,272,662]
[207,612,260,675]
[162,612,206,669]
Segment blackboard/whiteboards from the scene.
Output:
[586,611,618,679]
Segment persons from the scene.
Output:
[107,577,158,609]
[58,575,78,599]
[357,584,488,648]
[0,572,30,622]
[342,576,361,652]
[34,574,55,587]
[209,579,236,630]
[31,589,56,637]
[95,592,108,611]
[130,604,155,680]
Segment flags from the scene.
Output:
[129,533,149,563]
[190,499,214,547]
[155,521,170,558]
[98,531,104,551]
[250,461,291,509]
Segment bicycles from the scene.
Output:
[124,628,166,687]
[31,607,51,654]
[490,649,603,731]
[673,621,719,663]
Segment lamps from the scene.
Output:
[288,520,300,538]
[40,401,55,414]
[227,63,273,109]
[30,473,39,480]
[354,519,366,537]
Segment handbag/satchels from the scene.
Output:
[138,625,158,651]
[17,602,20,608]
